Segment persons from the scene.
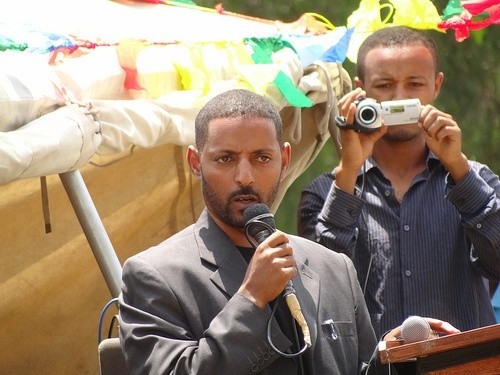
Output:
[293,25,500,356]
[113,88,467,375]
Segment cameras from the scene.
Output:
[346,97,422,135]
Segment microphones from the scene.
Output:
[403,316,444,344]
[242,204,314,351]
